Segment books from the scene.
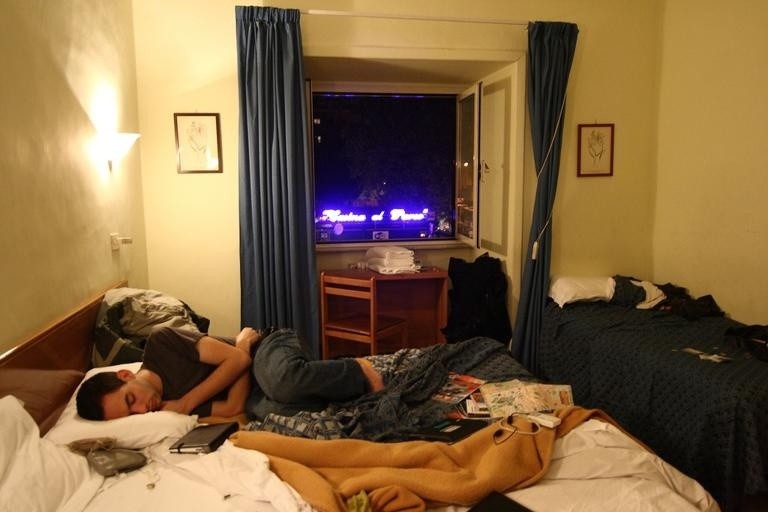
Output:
[169,423,239,454]
[415,372,575,442]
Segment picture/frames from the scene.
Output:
[174,113,223,174]
[577,124,614,177]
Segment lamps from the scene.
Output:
[107,133,139,171]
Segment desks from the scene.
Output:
[328,266,448,357]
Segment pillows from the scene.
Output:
[548,275,608,309]
[0,394,104,512]
[45,361,200,450]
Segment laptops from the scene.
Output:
[408,418,487,442]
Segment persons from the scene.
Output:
[77,327,385,423]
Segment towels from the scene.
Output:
[365,246,421,275]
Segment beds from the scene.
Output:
[0,280,721,512]
[539,299,768,512]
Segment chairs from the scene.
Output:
[320,271,408,359]
